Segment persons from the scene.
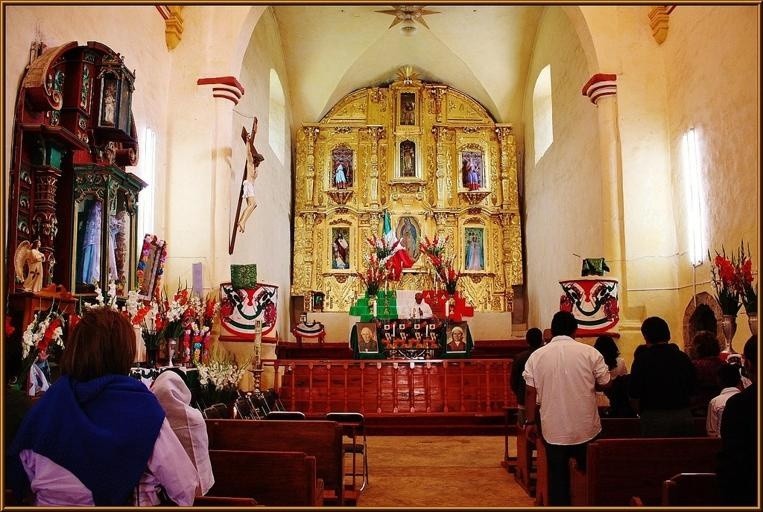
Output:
[18,309,199,506]
[466,235,483,269]
[398,216,418,264]
[102,82,119,127]
[689,332,741,410]
[15,239,45,294]
[83,202,124,284]
[401,99,415,126]
[523,310,611,508]
[237,133,258,233]
[359,327,377,351]
[399,143,414,169]
[332,229,349,271]
[509,327,545,481]
[706,336,758,509]
[591,335,629,383]
[630,317,699,441]
[462,156,480,185]
[410,292,432,322]
[446,327,465,350]
[335,164,348,189]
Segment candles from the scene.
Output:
[436,281,439,294]
[445,300,449,316]
[385,280,388,295]
[373,300,377,317]
[434,276,436,287]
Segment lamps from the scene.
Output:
[373,4,440,34]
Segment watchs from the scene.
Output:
[522,418,537,429]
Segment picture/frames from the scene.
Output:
[356,321,379,353]
[446,322,467,354]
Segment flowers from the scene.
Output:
[732,239,758,312]
[173,279,201,333]
[159,279,196,339]
[190,293,203,337]
[357,255,390,299]
[707,245,744,316]
[199,291,223,336]
[136,286,194,344]
[436,256,463,296]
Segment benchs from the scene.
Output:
[514,420,540,498]
[633,472,716,506]
[536,418,641,508]
[568,436,722,508]
[205,449,324,508]
[204,417,361,508]
[194,496,259,509]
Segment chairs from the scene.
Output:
[327,411,369,492]
[205,389,286,420]
[267,411,304,421]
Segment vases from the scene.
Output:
[369,294,377,315]
[722,316,737,353]
[748,314,758,333]
[162,338,178,368]
[191,337,202,368]
[449,298,455,314]
[201,332,209,362]
[178,330,192,368]
[145,344,160,369]
